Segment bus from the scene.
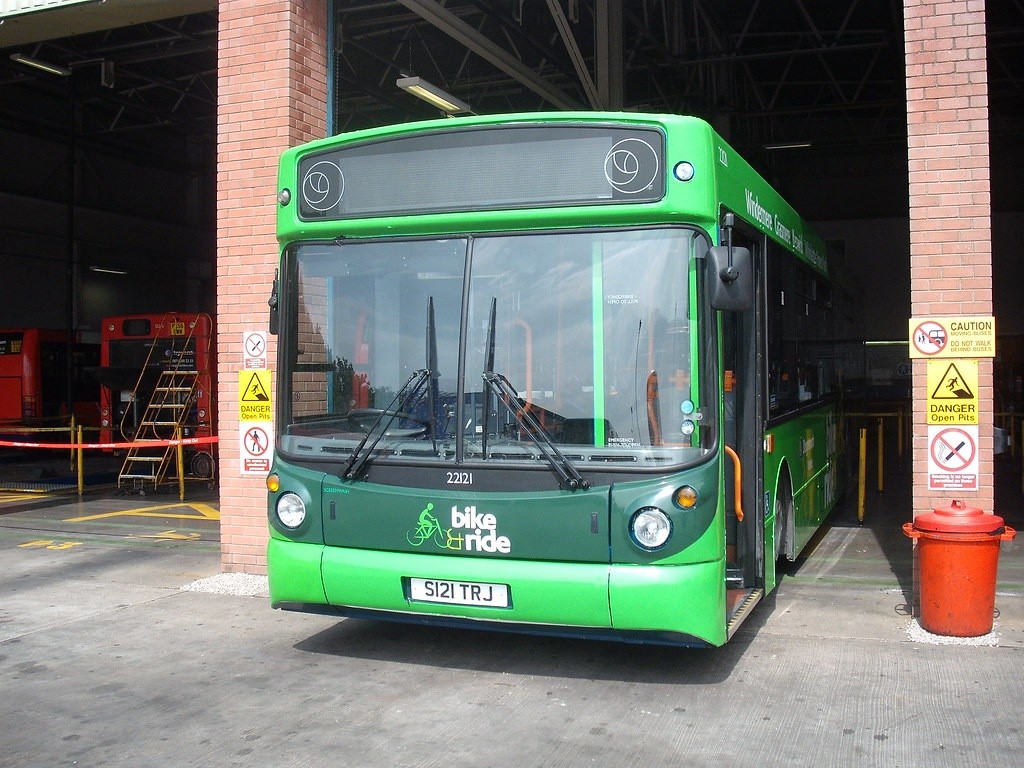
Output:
[98,312,217,480]
[0,328,103,459]
[264,106,870,652]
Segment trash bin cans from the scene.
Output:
[902,499,1017,637]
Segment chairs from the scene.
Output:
[405,357,455,439]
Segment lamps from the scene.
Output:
[395,10,470,114]
[10,53,73,77]
[762,140,813,150]
